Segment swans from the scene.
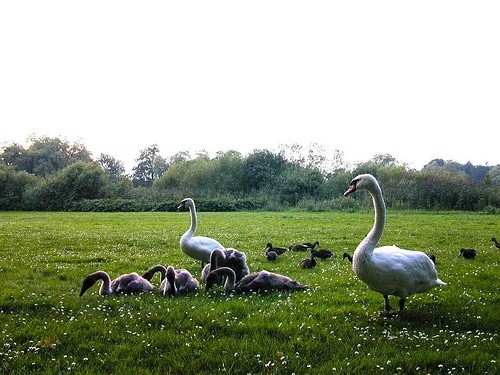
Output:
[80,198,331,297]
[459,248,477,259]
[490,238,500,249]
[342,172,448,316]
